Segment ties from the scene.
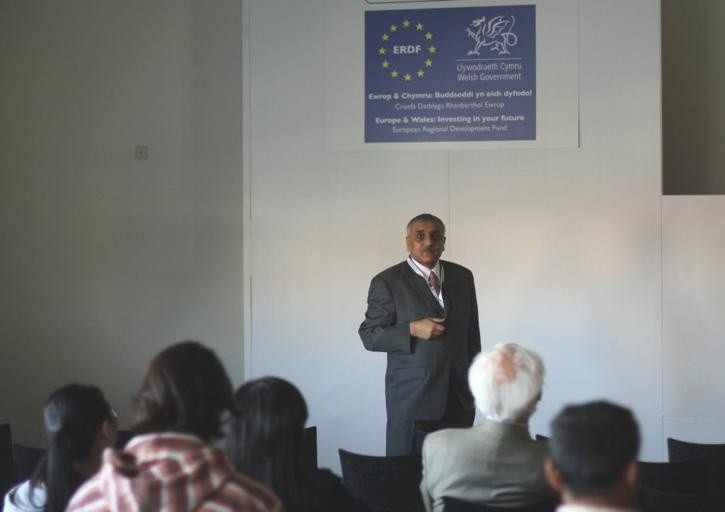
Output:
[428,271,442,300]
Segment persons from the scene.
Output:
[545,397,642,512]
[67,340,283,512]
[3,383,119,512]
[228,376,370,512]
[359,214,481,458]
[420,344,563,512]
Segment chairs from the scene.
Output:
[302,424,319,471]
[534,434,548,448]
[337,448,422,511]
[0,421,16,496]
[667,439,724,463]
